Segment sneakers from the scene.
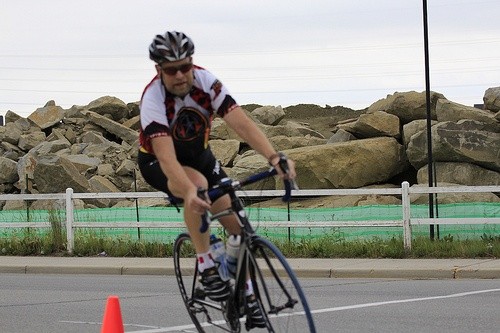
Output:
[245,294,266,328]
[197,267,231,299]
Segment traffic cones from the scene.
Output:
[100,295,125,333]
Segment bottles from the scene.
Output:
[209,234,230,282]
[225,232,241,275]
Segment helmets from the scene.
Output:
[150,31,194,63]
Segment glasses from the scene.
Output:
[160,61,193,76]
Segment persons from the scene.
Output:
[138,32,296,328]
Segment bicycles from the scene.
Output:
[163,152,315,333]
